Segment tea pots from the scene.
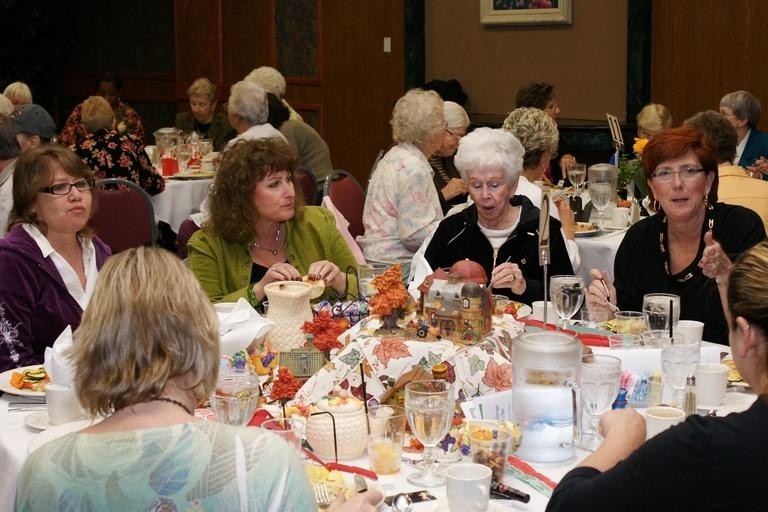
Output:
[262,278,317,356]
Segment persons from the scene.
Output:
[611,103,674,168]
[588,126,766,347]
[357,79,581,311]
[683,91,767,230]
[547,242,767,512]
[12,245,383,510]
[1,67,367,373]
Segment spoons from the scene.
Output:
[391,491,413,511]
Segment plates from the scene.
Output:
[23,410,48,429]
[0,364,52,401]
[367,481,385,512]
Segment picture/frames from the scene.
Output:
[476,0,572,28]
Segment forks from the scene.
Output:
[314,482,332,510]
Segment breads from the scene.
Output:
[301,276,325,299]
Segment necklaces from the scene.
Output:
[141,396,194,416]
[658,204,714,282]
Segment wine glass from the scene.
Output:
[404,378,457,487]
[567,162,617,235]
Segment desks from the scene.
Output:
[1,147,767,512]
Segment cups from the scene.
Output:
[263,300,269,315]
[533,180,543,188]
[214,302,236,312]
[363,402,409,472]
[45,383,80,425]
[210,368,260,427]
[610,207,630,227]
[357,263,391,300]
[144,127,220,179]
[444,461,493,511]
[262,417,305,456]
[465,425,512,487]
[512,273,730,464]
[491,294,507,316]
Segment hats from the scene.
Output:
[7,104,56,140]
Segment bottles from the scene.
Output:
[630,195,642,224]
[432,364,449,381]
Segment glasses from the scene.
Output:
[546,103,559,112]
[38,177,95,196]
[650,166,705,182]
[721,111,737,119]
[446,130,461,143]
